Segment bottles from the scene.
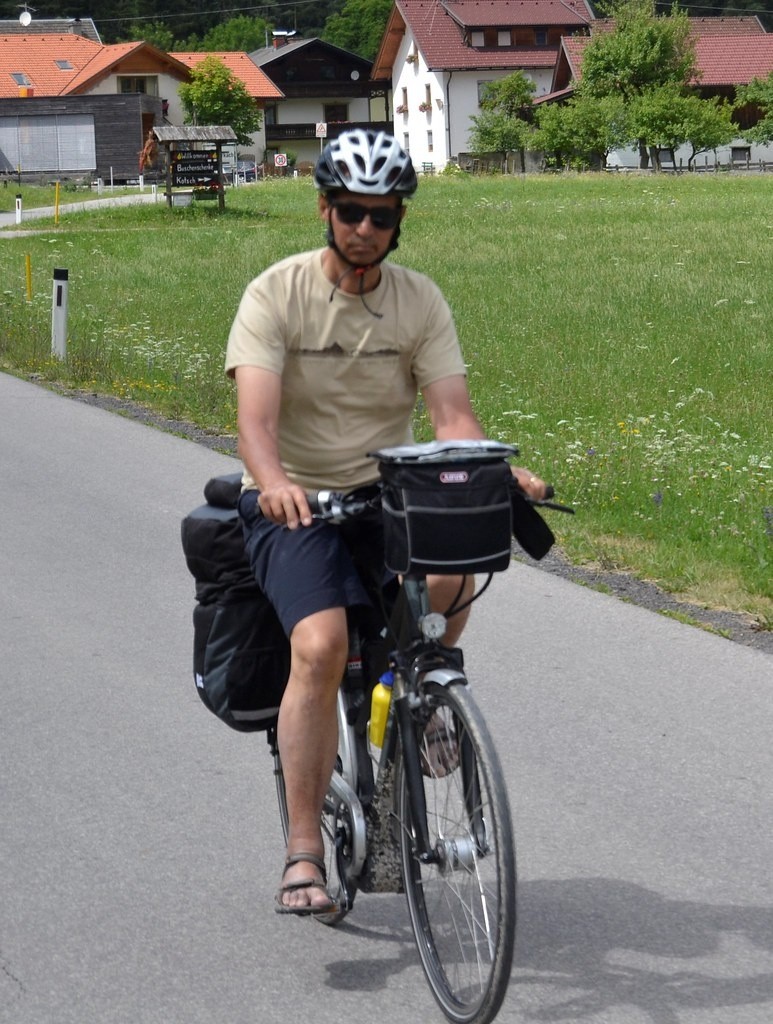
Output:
[348,606,362,670]
[370,671,394,763]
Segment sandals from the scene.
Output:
[413,715,465,778]
[275,853,336,914]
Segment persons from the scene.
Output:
[224,129,545,917]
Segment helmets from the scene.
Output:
[314,128,419,198]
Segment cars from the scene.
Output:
[221,160,257,185]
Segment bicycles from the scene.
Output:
[251,472,577,1024]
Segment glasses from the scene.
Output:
[324,197,404,230]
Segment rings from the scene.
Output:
[531,477,537,481]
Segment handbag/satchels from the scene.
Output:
[179,472,431,732]
